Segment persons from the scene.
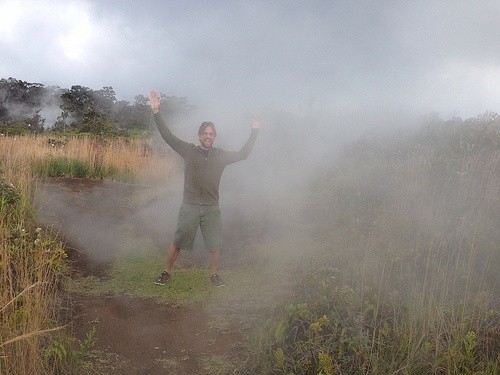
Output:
[148,88,262,287]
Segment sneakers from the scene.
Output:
[208,272,224,288]
[153,270,171,286]
